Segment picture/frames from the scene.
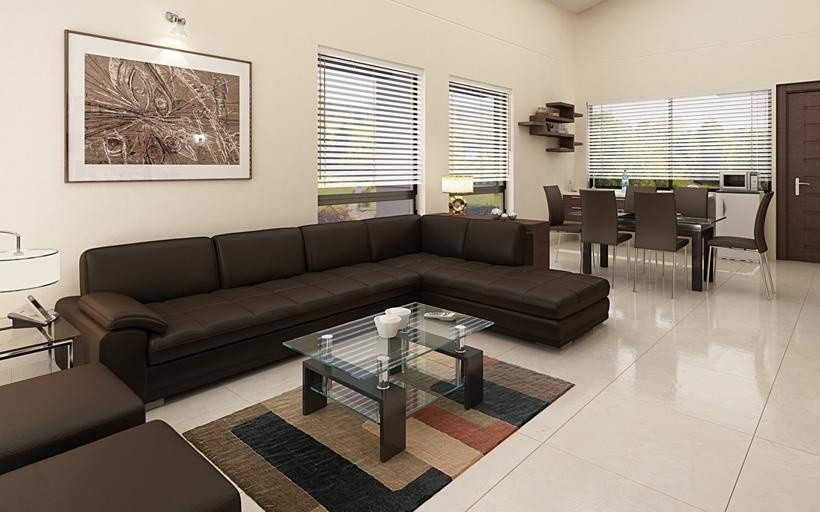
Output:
[63,28,252,184]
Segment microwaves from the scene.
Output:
[719,170,757,191]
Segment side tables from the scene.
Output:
[0,308,82,369]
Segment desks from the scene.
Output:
[473,218,550,269]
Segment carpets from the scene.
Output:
[182,343,575,512]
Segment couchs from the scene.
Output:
[53,212,610,412]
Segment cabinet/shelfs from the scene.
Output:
[518,102,583,153]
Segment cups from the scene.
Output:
[373,315,400,338]
[384,306,411,331]
[761,180,771,192]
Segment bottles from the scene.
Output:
[536,107,548,116]
[568,180,572,192]
[622,170,628,191]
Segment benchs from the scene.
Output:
[0,362,146,475]
[0,420,241,512]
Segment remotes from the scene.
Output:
[424,312,455,321]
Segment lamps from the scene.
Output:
[440,174,474,215]
[164,11,189,40]
[0,230,61,293]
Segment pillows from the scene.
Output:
[80,237,220,303]
[300,220,371,272]
[366,214,421,262]
[463,218,524,267]
[214,227,306,288]
[421,212,470,258]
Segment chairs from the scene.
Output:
[543,184,776,300]
